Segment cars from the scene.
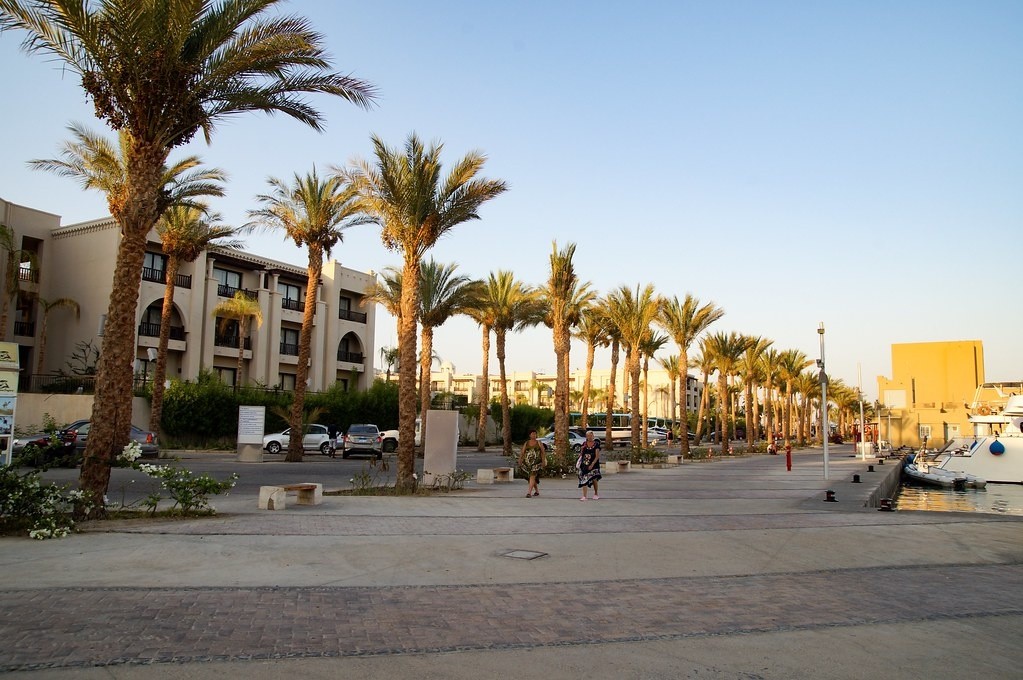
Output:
[703,431,735,442]
[536,431,586,453]
[736,429,746,440]
[647,425,697,443]
[262,423,345,456]
[11,416,161,461]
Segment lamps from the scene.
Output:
[97,314,106,337]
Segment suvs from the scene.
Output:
[380,418,460,453]
[341,424,385,460]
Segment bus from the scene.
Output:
[569,412,642,448]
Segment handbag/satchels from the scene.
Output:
[538,441,547,466]
[576,441,587,469]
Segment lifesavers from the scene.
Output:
[977,406,991,416]
[864,426,871,435]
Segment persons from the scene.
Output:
[667,428,673,449]
[518,429,545,498]
[844,434,848,440]
[578,430,602,500]
[327,421,341,459]
[766,441,779,456]
[864,426,873,442]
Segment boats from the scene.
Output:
[911,381,1023,486]
[901,460,988,492]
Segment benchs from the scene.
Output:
[605,460,631,474]
[476,466,514,484]
[258,482,323,511]
[668,454,684,465]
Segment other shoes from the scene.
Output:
[533,492,539,496]
[593,495,599,500]
[580,497,588,501]
[526,494,531,498]
